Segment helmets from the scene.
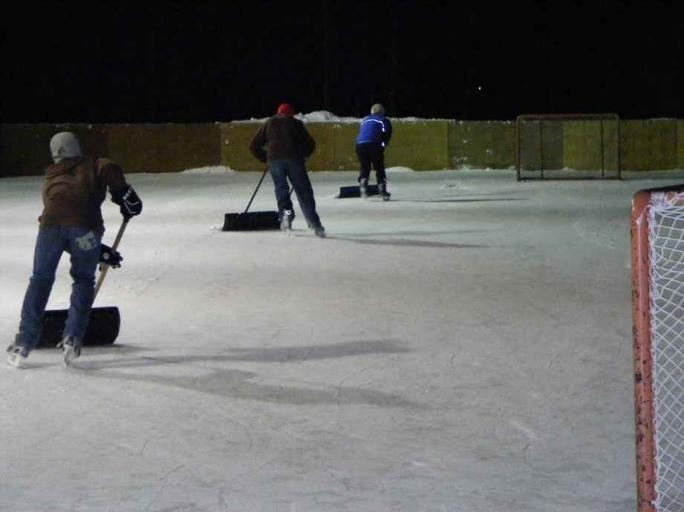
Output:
[49,132,82,166]
[371,103,385,115]
[277,103,294,116]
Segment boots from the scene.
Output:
[358,179,369,198]
[378,183,390,197]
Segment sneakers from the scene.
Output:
[276,208,295,223]
[7,337,32,358]
[312,224,324,236]
[58,336,82,359]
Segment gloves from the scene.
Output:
[97,243,124,272]
[111,185,143,220]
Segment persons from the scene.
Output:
[354,102,393,197]
[248,102,325,234]
[5,130,143,360]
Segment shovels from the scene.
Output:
[37,214,129,348]
[223,163,280,230]
[340,185,379,198]
